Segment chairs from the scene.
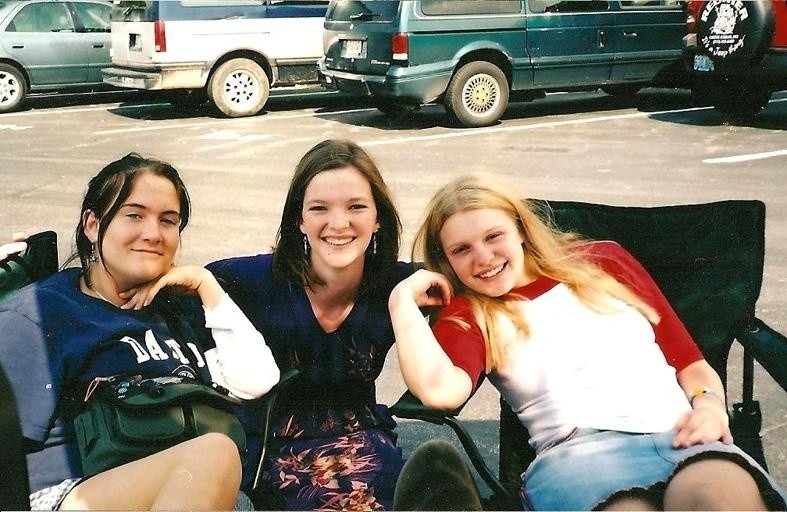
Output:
[0,231,304,511]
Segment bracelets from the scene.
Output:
[688,387,726,410]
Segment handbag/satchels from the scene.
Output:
[74,382,247,478]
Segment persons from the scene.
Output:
[0,139,486,512]
[0,153,281,512]
